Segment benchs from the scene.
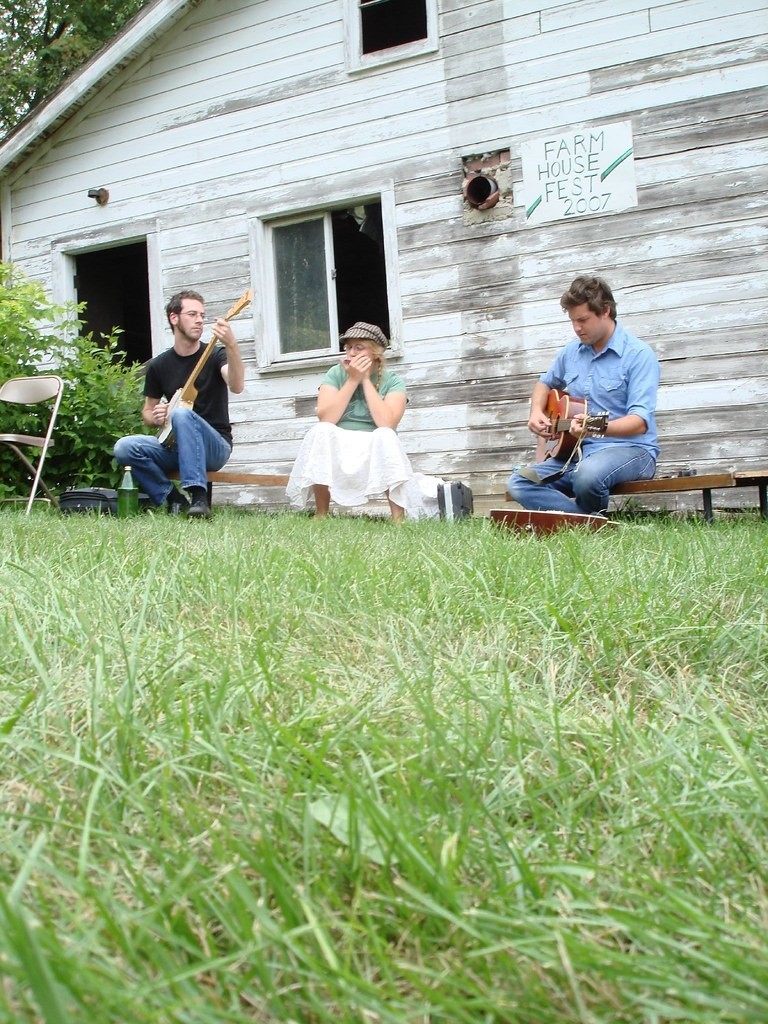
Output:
[166,470,290,518]
[504,469,768,523]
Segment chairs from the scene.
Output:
[0,374,63,518]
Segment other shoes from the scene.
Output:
[186,498,210,520]
[168,494,190,518]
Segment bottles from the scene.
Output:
[118,465,140,519]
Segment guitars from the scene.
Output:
[159,286,254,447]
[535,389,610,461]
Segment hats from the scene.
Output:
[339,321,388,352]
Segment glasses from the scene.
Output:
[174,311,205,319]
[343,343,373,352]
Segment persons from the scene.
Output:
[114,290,244,519]
[285,322,410,525]
[507,275,662,518]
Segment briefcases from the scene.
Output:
[437,481,474,522]
